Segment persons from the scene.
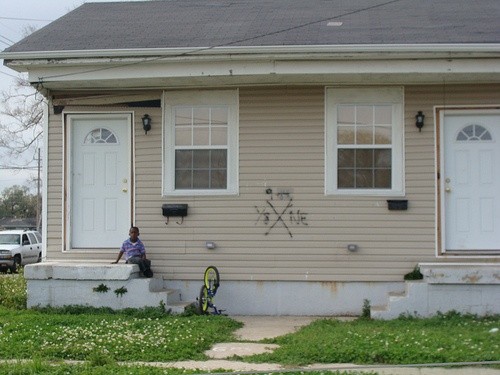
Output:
[111,227,154,278]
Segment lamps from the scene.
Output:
[140,113,153,136]
[415,110,426,132]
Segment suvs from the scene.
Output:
[0,229,43,271]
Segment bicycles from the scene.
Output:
[196,264,223,314]
